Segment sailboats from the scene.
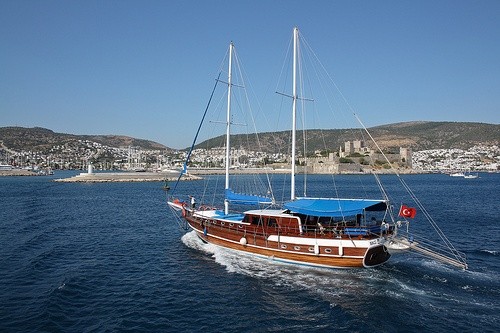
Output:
[163,26,469,271]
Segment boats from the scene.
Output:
[463,173,479,179]
[449,171,464,177]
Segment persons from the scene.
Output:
[188,195,196,208]
[360,215,365,226]
[381,219,389,234]
[371,217,376,225]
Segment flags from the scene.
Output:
[399,205,417,219]
